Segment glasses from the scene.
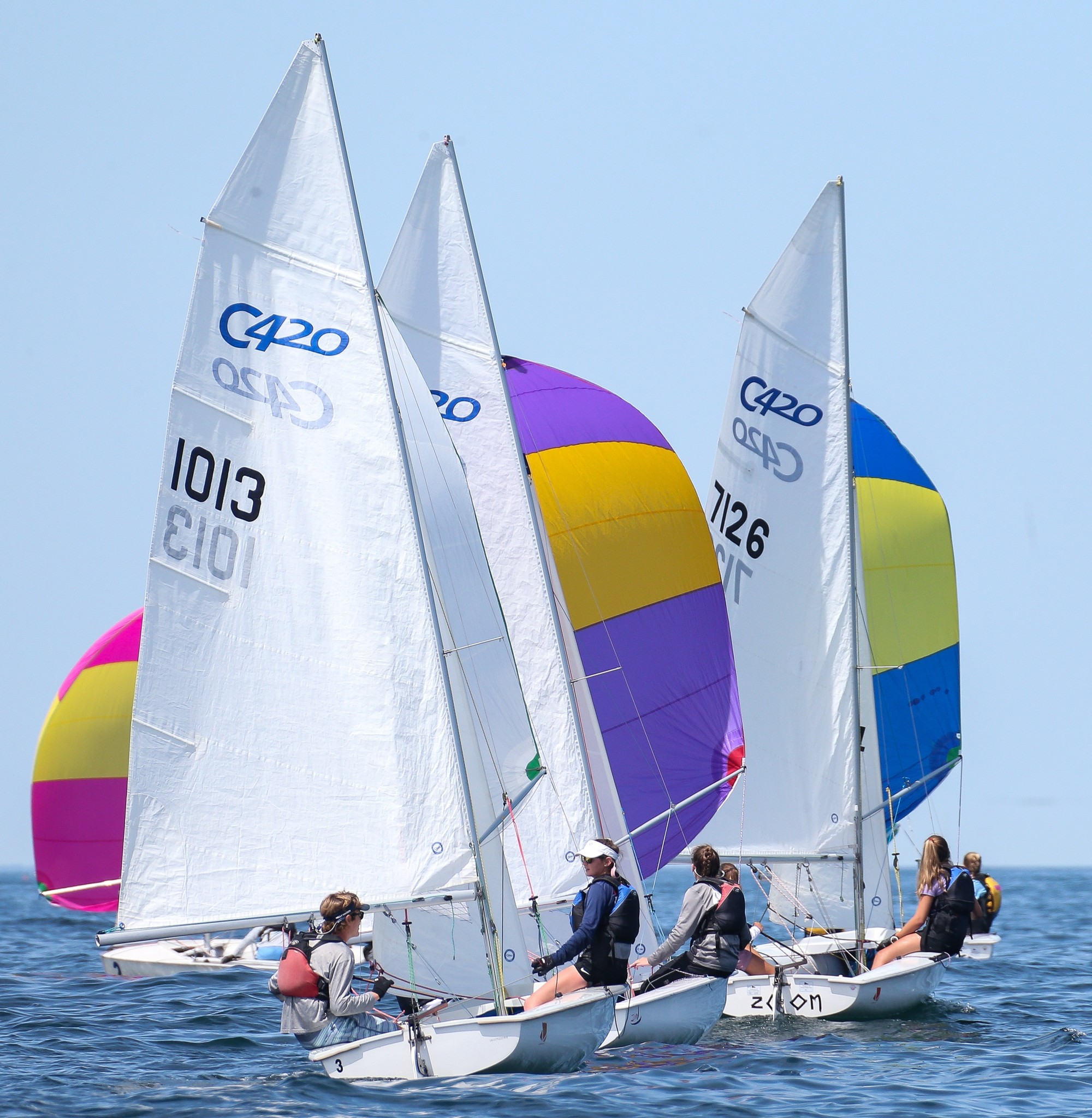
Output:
[356,912,364,920]
[581,855,592,864]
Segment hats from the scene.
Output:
[316,901,369,931]
[574,840,619,860]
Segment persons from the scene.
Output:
[720,862,775,975]
[962,851,1002,933]
[524,839,641,1014]
[630,844,752,994]
[255,921,295,960]
[268,891,408,1049]
[870,834,983,970]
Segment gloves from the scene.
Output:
[531,955,554,977]
[371,975,395,1002]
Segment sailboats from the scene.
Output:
[663,173,947,1025]
[850,397,1005,966]
[381,143,752,1049]
[91,26,634,1079]
